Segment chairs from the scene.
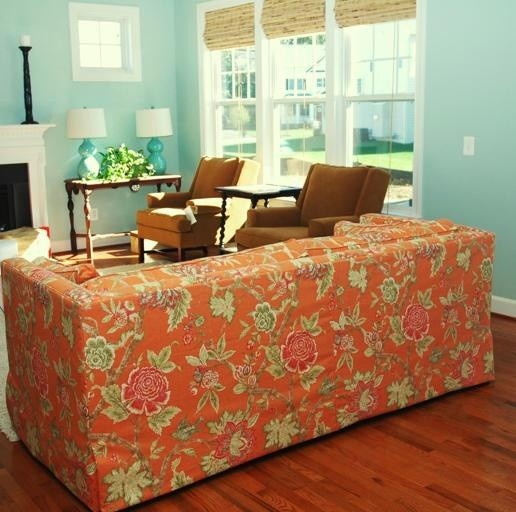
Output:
[233,163,391,253]
[136,155,245,264]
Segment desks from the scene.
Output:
[63,174,182,264]
[212,183,304,255]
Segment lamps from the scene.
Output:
[65,106,108,180]
[135,105,173,175]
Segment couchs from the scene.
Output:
[0,212,496,512]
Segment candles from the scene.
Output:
[20,34,31,46]
